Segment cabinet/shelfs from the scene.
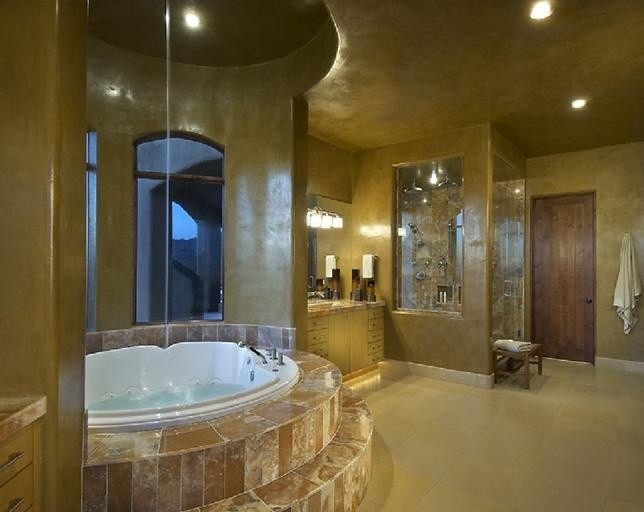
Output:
[369,308,385,364]
[306,314,329,363]
[328,310,370,376]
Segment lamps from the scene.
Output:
[307,206,344,230]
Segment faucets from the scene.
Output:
[314,290,325,300]
[238,341,267,366]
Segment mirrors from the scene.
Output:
[306,192,353,302]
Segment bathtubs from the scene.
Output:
[85,341,299,431]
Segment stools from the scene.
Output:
[493,343,543,389]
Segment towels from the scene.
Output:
[613,232,643,336]
[362,254,374,278]
[495,339,532,353]
[325,255,336,277]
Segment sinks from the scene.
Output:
[311,303,341,308]
